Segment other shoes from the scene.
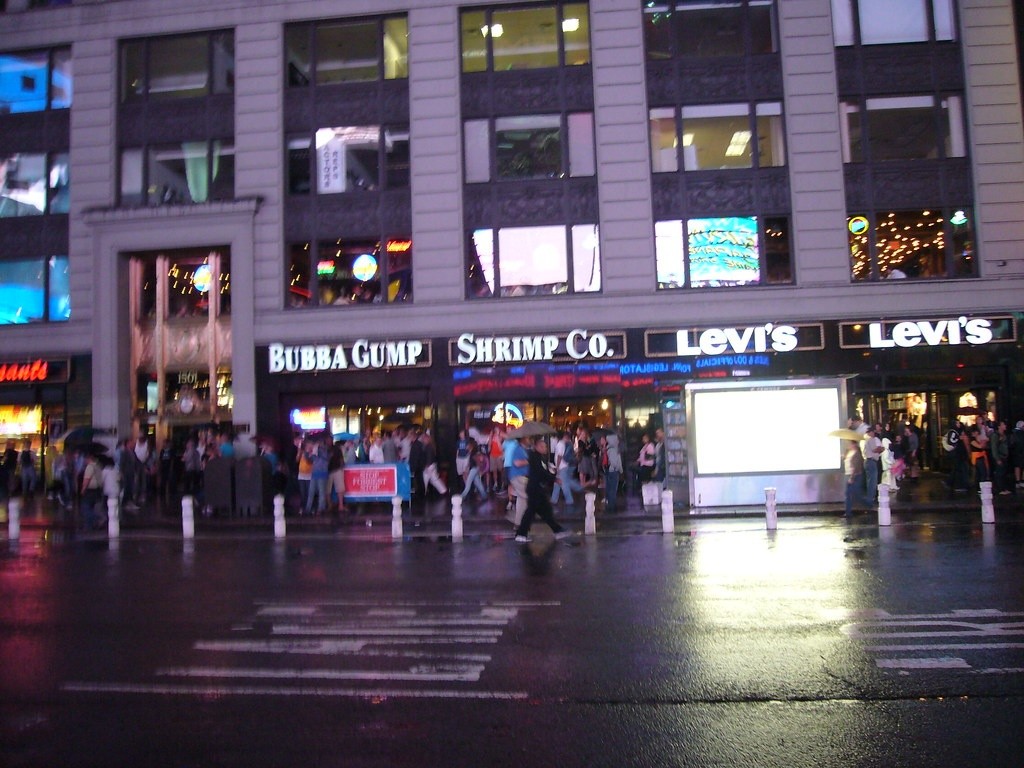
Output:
[507,502,513,510]
[999,490,1011,494]
[555,531,572,540]
[514,535,532,542]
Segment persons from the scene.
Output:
[841,414,1024,518]
[0,418,665,542]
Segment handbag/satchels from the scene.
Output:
[85,487,100,499]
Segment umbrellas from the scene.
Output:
[508,421,557,444]
[829,428,865,441]
